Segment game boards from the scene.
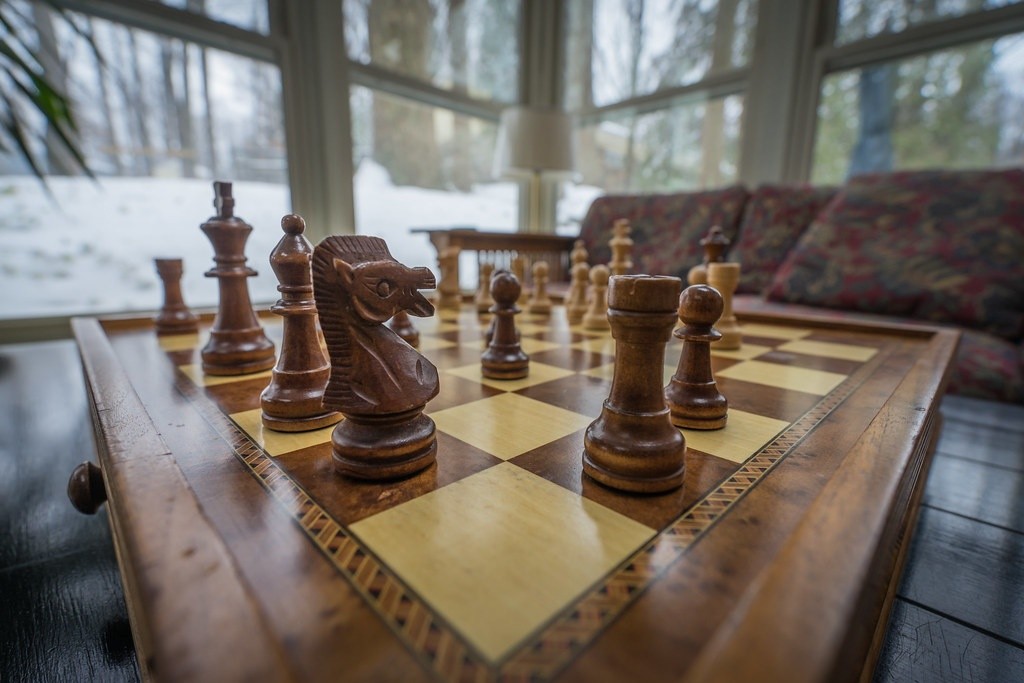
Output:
[70,289,965,683]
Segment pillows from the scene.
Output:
[761,167,1024,335]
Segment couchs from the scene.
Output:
[408,165,1024,408]
